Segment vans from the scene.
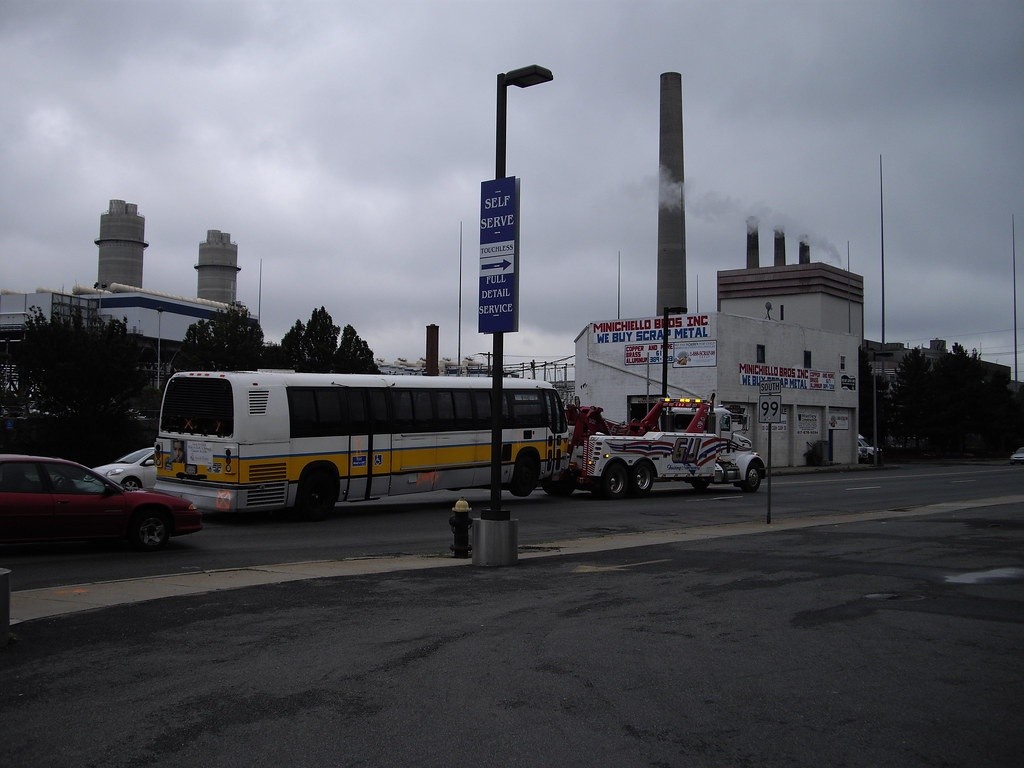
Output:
[858,433,883,462]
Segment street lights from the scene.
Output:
[480,63,556,519]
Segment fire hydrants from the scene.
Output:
[447,497,473,559]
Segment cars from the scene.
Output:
[80,447,158,490]
[0,455,205,553]
[1010,447,1024,465]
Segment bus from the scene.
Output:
[154,368,569,524]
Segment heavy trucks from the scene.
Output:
[564,392,769,500]
